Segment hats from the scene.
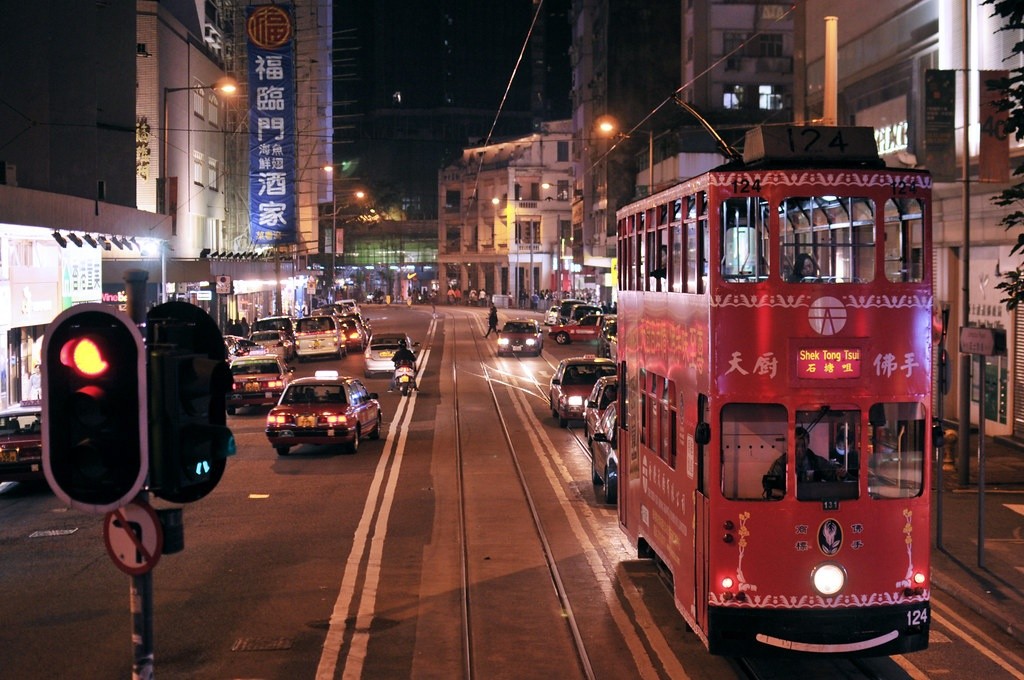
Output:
[836,428,854,443]
[795,427,809,438]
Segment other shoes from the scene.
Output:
[483,336,487,338]
[414,388,420,392]
[387,389,393,392]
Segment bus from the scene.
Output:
[615,90,941,657]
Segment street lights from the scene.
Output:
[599,121,654,196]
[159,78,239,217]
[491,196,522,310]
[332,190,365,292]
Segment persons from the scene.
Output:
[602,386,616,409]
[28,364,41,398]
[597,301,617,313]
[651,245,667,279]
[768,427,836,499]
[407,286,422,304]
[482,303,497,339]
[531,288,592,311]
[446,288,461,305]
[225,317,258,337]
[387,341,420,392]
[788,253,820,282]
[831,430,858,479]
[468,288,486,306]
[301,295,324,316]
[521,289,529,310]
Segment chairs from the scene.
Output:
[0,419,41,432]
[294,387,319,402]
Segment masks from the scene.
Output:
[836,446,849,455]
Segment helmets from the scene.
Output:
[398,340,407,349]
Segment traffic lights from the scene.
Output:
[38,302,149,514]
[140,298,231,504]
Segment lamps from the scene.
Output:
[51,229,68,248]
[110,233,123,250]
[200,248,293,259]
[82,232,97,248]
[119,235,133,250]
[67,230,83,247]
[130,237,140,249]
[96,234,112,250]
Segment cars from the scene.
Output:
[583,375,618,449]
[546,313,612,344]
[220,333,268,361]
[362,332,421,377]
[258,298,373,360]
[549,353,617,428]
[495,319,547,358]
[226,353,296,415]
[367,290,384,305]
[263,369,382,456]
[248,330,297,364]
[543,298,602,325]
[589,399,619,505]
[0,399,43,492]
[597,313,620,361]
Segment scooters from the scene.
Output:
[393,351,419,397]
